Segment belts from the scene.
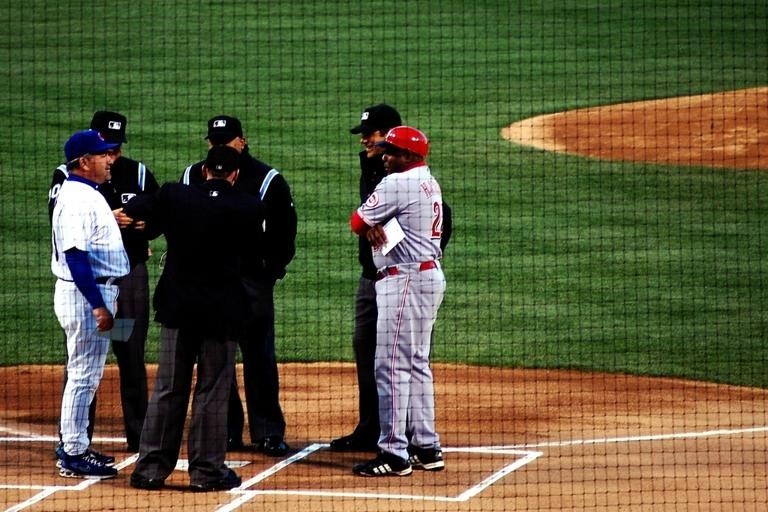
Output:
[377,260,437,279]
[94,276,124,287]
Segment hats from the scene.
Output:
[349,103,402,134]
[207,144,244,174]
[89,111,128,144]
[204,116,242,141]
[64,130,119,165]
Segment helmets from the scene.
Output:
[374,126,431,160]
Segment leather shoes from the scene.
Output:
[126,432,378,491]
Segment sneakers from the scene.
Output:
[407,445,444,471]
[353,447,413,477]
[57,448,118,480]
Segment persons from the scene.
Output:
[39,110,160,452]
[50,131,130,477]
[124,142,248,492]
[350,124,445,477]
[181,114,296,455]
[331,102,452,449]
